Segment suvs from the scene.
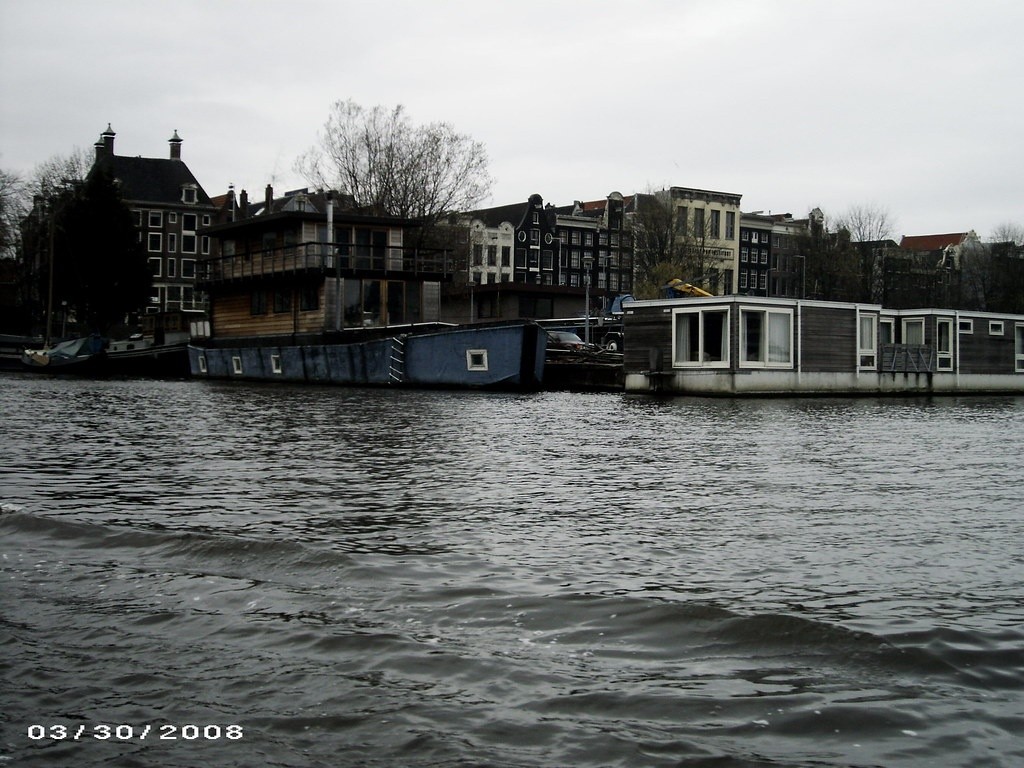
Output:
[602,324,624,351]
[547,331,594,361]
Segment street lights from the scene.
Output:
[581,255,595,346]
[603,256,612,309]
[794,255,806,300]
[765,268,776,297]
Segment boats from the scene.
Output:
[186,321,550,395]
[0,332,210,377]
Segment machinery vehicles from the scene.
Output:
[668,279,714,297]
